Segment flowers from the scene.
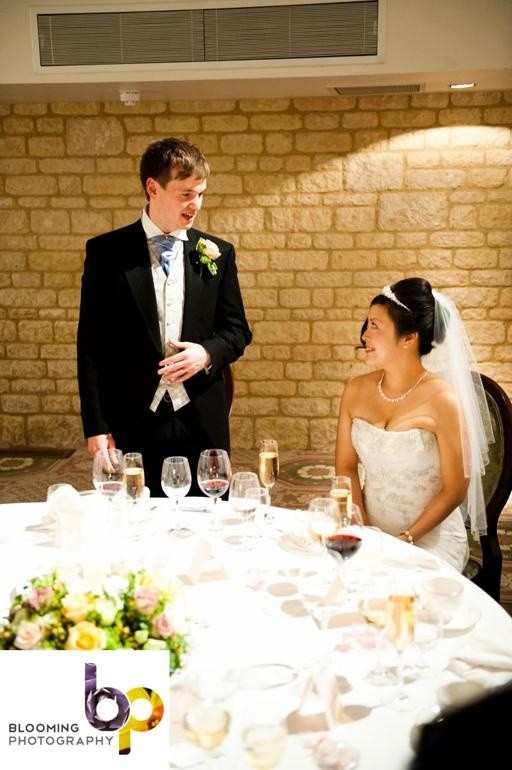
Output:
[0,567,188,679]
[196,236,221,276]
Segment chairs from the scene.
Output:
[435,371,512,612]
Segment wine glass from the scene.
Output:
[47,440,288,552]
[310,475,364,598]
[384,592,443,703]
[178,703,368,770]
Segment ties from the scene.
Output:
[152,237,178,278]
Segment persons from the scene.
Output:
[341,275,494,583]
[75,137,257,505]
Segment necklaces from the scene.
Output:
[377,366,426,406]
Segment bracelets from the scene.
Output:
[401,527,415,547]
